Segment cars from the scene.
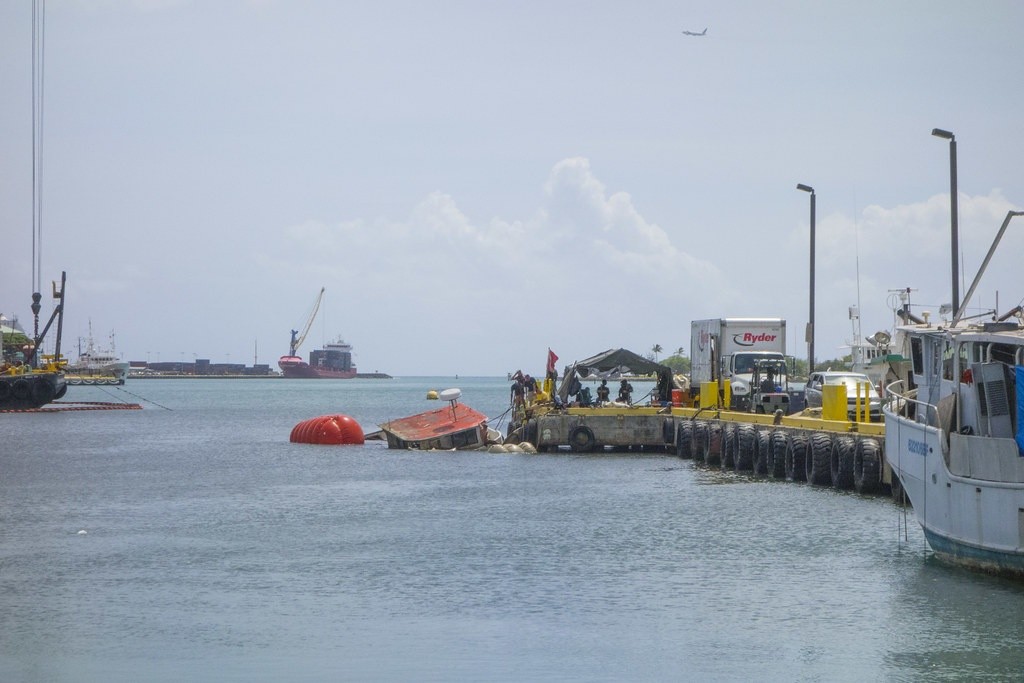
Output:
[804,371,881,422]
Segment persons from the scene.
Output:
[511,369,525,383]
[524,374,540,407]
[510,378,526,410]
[615,380,633,404]
[596,379,610,402]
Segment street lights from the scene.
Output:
[796,182,816,372]
[932,128,958,319]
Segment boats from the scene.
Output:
[40,319,129,385]
[365,389,504,453]
[0,270,69,410]
[278,286,357,379]
[884,211,1024,578]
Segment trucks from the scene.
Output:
[691,318,793,410]
